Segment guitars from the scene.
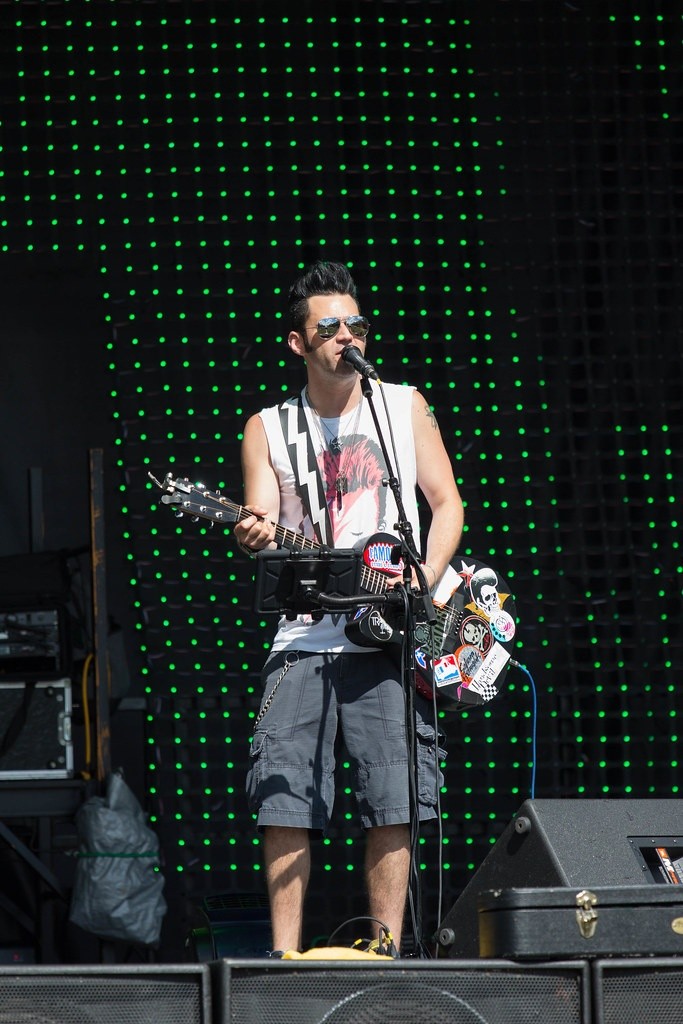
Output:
[147,470,519,714]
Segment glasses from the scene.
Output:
[304,315,371,340]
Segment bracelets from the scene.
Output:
[426,564,436,593]
[239,542,266,554]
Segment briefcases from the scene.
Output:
[475,883,683,961]
[0,675,76,782]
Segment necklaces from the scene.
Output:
[306,391,361,451]
[305,391,363,510]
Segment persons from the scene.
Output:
[235,261,464,960]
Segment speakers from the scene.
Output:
[0,962,209,1024]
[588,958,683,1024]
[436,799,683,962]
[218,955,594,1024]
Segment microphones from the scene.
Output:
[340,345,380,381]
